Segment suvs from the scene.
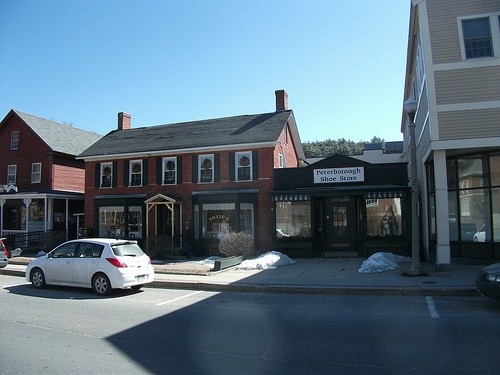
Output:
[461,223,477,240]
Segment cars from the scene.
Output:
[474,262,500,300]
[473,224,486,242]
[26,238,154,296]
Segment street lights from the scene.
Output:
[404,95,426,265]
